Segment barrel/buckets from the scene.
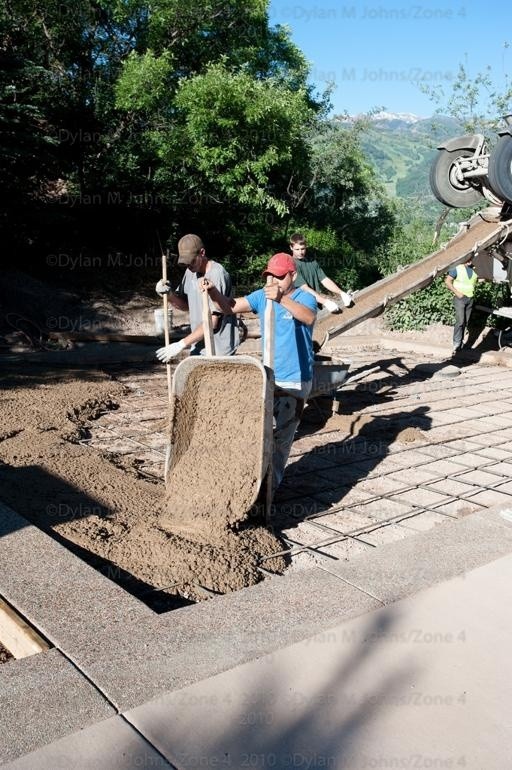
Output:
[153,308,174,333]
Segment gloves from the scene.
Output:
[339,291,354,308]
[154,277,173,299]
[323,298,341,314]
[154,340,187,363]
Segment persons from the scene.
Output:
[198,252,318,503]
[289,232,352,314]
[444,262,479,360]
[155,234,241,364]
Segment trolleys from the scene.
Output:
[154,255,280,519]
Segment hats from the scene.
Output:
[176,232,204,266]
[261,250,297,279]
